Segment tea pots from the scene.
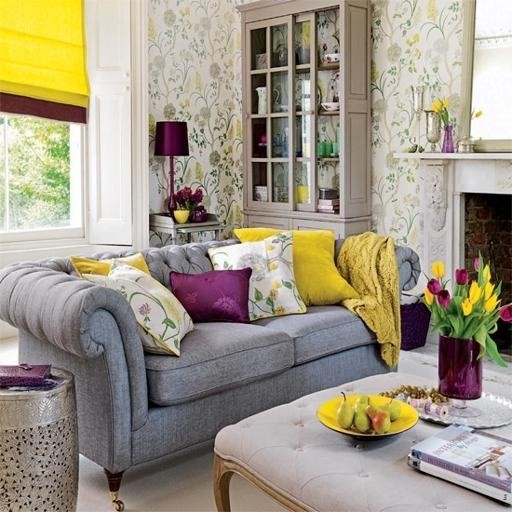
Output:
[256,87,279,117]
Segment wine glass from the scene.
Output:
[423,108,444,153]
[409,84,423,155]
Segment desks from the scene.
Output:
[149,212,225,245]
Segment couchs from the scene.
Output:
[0,238,421,512]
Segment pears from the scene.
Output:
[337,392,400,435]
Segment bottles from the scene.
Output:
[281,127,289,158]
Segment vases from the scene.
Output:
[442,126,454,153]
[400,301,432,351]
[438,336,483,399]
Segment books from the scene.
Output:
[317,199,340,214]
[0,364,51,385]
[407,423,511,505]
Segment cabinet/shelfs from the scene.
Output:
[235,0,371,220]
[237,209,373,241]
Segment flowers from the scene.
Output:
[431,96,459,127]
[422,249,512,369]
[470,105,482,123]
[175,187,206,222]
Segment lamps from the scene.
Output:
[154,122,189,224]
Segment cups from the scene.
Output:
[324,53,340,63]
[318,143,333,158]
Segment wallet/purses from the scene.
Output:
[0,364,57,386]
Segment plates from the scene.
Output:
[317,393,420,444]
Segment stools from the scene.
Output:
[0,366,79,512]
[212,371,511,512]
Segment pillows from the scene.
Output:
[68,228,360,357]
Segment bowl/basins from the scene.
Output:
[321,102,340,111]
[280,105,299,112]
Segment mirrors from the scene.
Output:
[453,0,512,153]
